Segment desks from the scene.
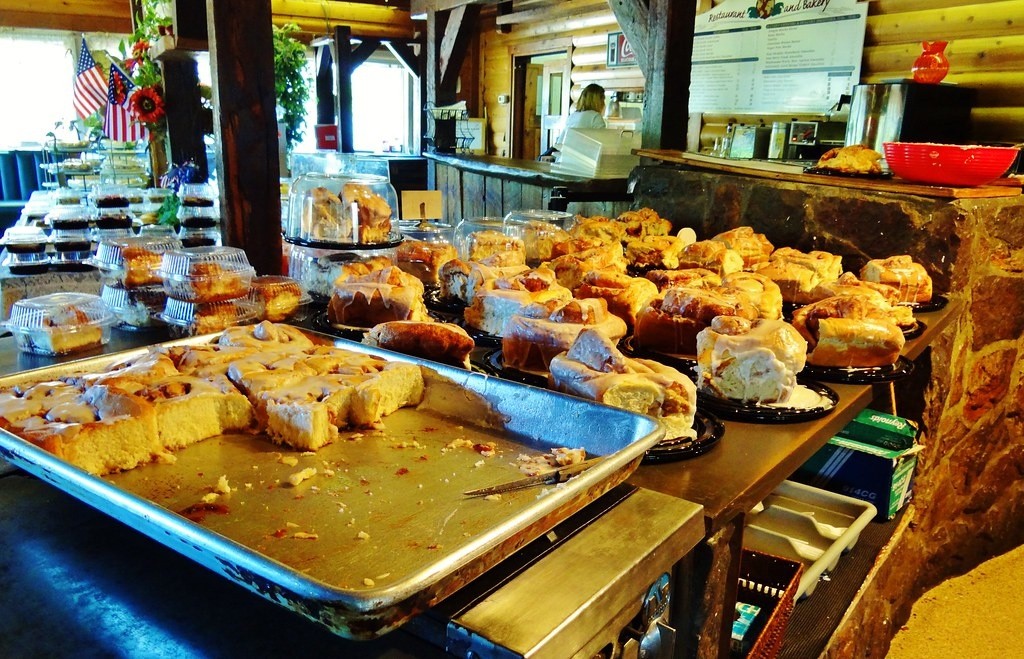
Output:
[0,287,962,648]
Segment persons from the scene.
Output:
[534,83,607,162]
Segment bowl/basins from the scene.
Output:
[882,141,1022,187]
[0,237,316,358]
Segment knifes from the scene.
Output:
[463,454,609,496]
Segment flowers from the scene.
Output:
[113,1,174,148]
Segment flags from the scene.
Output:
[103,64,152,142]
[73,38,109,120]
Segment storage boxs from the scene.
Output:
[726,406,929,659]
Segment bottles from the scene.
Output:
[720,126,733,157]
[610,41,615,62]
[767,121,786,158]
[911,40,949,84]
[710,137,721,156]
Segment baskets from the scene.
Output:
[723,549,806,659]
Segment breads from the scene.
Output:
[0,183,935,476]
[817,144,885,174]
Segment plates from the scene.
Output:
[802,165,897,179]
[307,281,951,463]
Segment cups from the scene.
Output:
[624,92,643,102]
